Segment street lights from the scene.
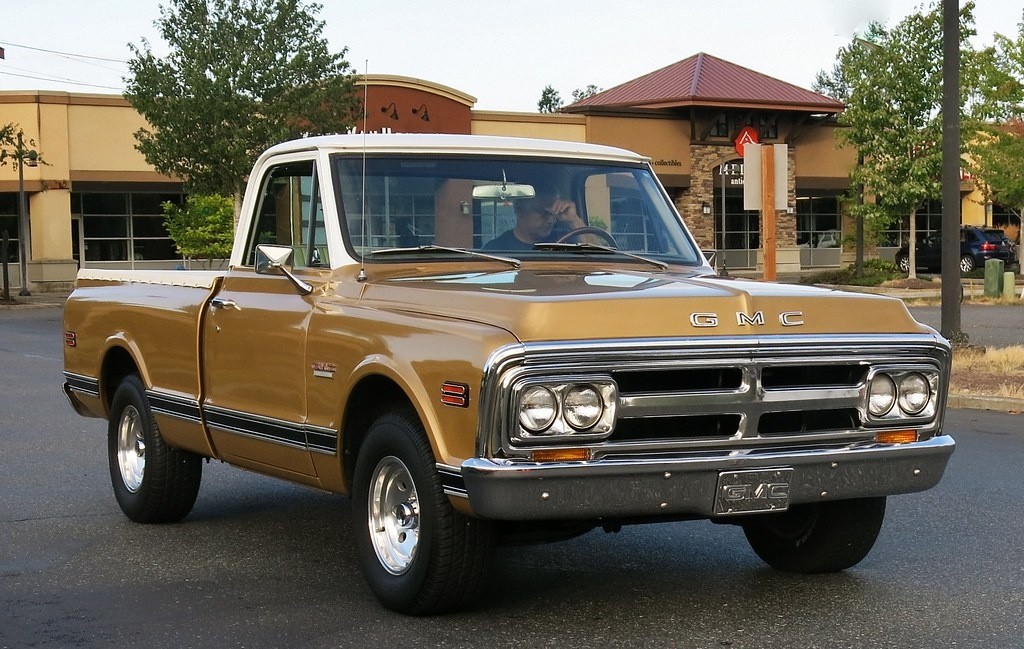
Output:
[0,132,37,296]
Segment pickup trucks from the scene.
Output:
[61,134,956,616]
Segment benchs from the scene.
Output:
[293,244,395,268]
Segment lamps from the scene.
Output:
[702,202,712,215]
[381,103,398,120]
[412,104,429,121]
[460,200,470,215]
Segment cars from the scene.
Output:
[895,224,1019,273]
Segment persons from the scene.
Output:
[482,180,607,253]
